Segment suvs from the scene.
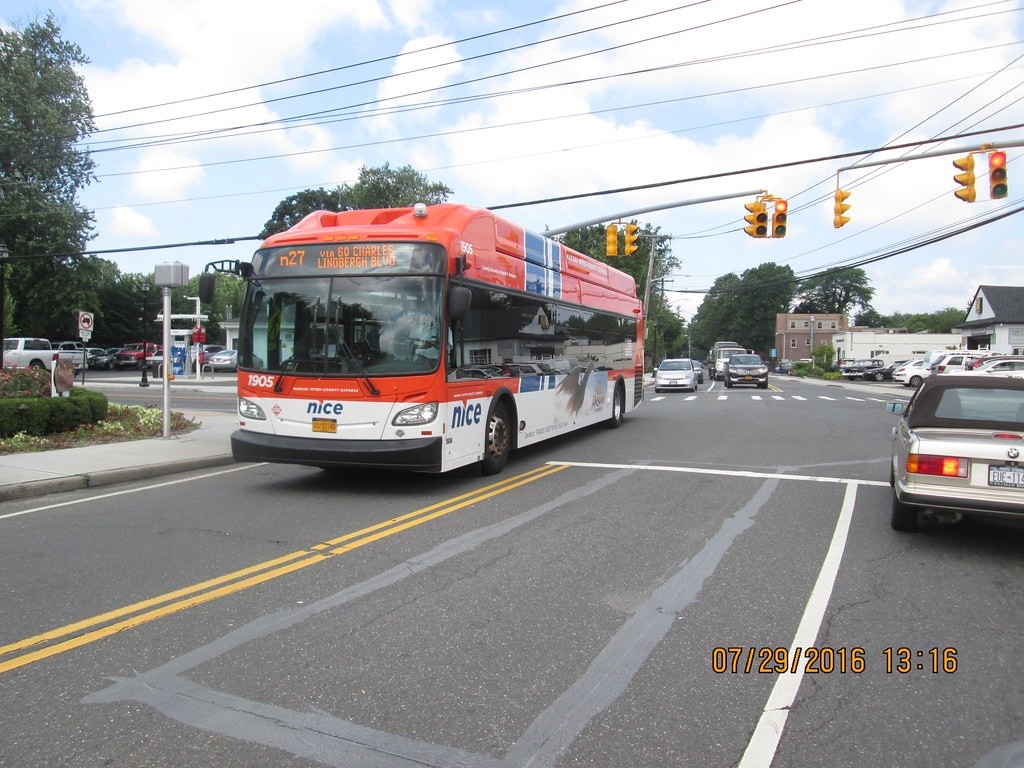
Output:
[723,354,769,389]
[931,354,983,374]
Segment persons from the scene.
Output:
[390,301,453,359]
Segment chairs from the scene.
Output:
[935,389,961,419]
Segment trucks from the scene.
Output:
[924,350,1004,377]
[708,342,756,381]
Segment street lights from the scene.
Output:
[646,274,692,338]
[139,279,152,387]
[776,332,785,359]
[651,323,657,378]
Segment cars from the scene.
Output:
[654,357,704,393]
[774,361,795,376]
[51,341,263,372]
[838,350,1024,533]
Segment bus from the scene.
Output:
[198,202,645,477]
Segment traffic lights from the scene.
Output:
[772,200,788,238]
[989,152,1008,199]
[756,202,768,238]
[953,157,976,203]
[834,190,851,229]
[625,224,640,257]
[743,203,756,238]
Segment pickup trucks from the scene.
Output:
[3,338,89,378]
